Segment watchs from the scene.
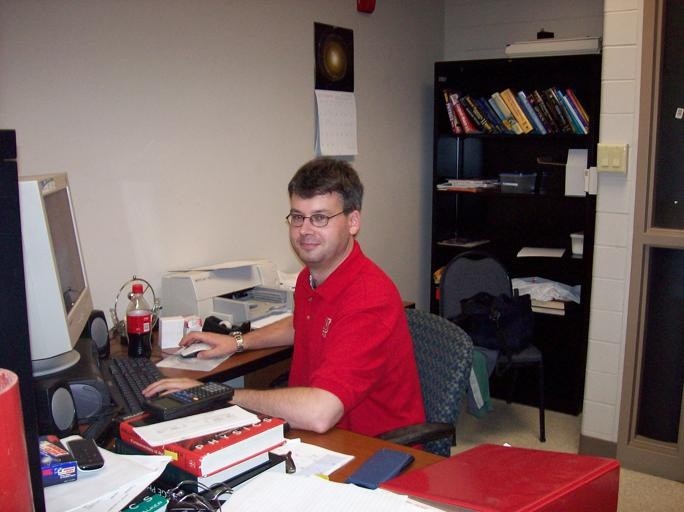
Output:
[230,331,245,353]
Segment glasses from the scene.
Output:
[285,211,345,228]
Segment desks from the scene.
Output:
[33,301,449,483]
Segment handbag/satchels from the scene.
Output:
[448,288,534,354]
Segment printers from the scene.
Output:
[162,259,296,328]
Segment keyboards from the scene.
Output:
[102,355,168,418]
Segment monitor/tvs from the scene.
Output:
[18,171,93,375]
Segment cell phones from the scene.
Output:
[67,439,102,470]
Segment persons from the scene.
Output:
[140,158,431,453]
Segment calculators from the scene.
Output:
[145,380,234,419]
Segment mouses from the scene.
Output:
[178,343,212,357]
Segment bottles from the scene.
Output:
[125,284,153,361]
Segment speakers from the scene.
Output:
[84,310,109,364]
[20,379,77,443]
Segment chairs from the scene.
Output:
[374,308,474,457]
[440,251,546,442]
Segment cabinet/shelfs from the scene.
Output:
[430,54,602,417]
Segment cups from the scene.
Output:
[0,369,36,512]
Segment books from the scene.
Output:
[113,402,288,494]
[436,176,499,193]
[443,86,591,137]
[437,238,490,249]
[530,297,567,316]
[379,443,620,512]
